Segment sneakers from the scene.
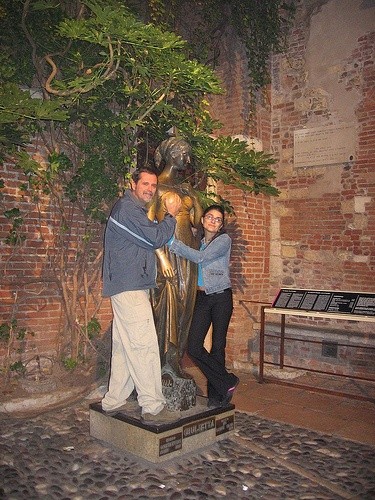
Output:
[207,373,240,408]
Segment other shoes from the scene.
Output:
[144,406,167,421]
[109,404,127,413]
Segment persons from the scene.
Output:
[164,205,240,408]
[144,136,203,364]
[102,165,183,425]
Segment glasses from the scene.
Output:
[205,214,223,223]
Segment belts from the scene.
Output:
[197,291,216,296]
